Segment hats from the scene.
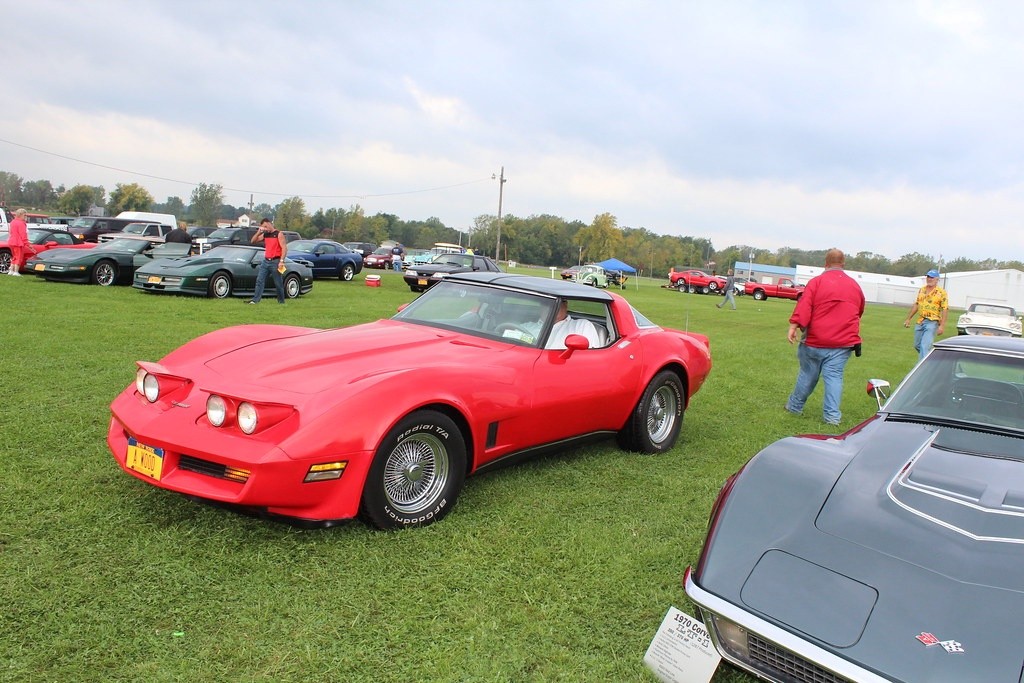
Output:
[925,269,941,278]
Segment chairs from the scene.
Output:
[593,322,607,347]
[950,378,1023,419]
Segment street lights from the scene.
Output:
[578,246,584,266]
[468,233,473,247]
[491,166,507,266]
[748,249,755,281]
[459,227,463,246]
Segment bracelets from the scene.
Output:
[280,260,284,262]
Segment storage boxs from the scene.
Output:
[365,275,381,287]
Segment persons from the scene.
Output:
[165,222,192,255]
[717,269,736,309]
[515,300,601,349]
[904,270,948,362]
[248,218,287,304]
[465,247,481,255]
[391,243,404,271]
[7,209,31,277]
[782,249,865,426]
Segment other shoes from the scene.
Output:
[716,304,722,308]
[244,299,259,304]
[8,271,23,276]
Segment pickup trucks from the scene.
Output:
[745,276,806,301]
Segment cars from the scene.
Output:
[404,253,505,294]
[432,248,461,254]
[717,275,757,295]
[412,252,442,265]
[561,265,624,288]
[0,205,408,281]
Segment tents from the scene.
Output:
[591,258,639,290]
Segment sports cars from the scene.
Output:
[0,228,102,275]
[957,302,1023,337]
[682,335,1024,683]
[671,270,727,290]
[23,238,193,286]
[131,244,315,300]
[104,270,713,532]
